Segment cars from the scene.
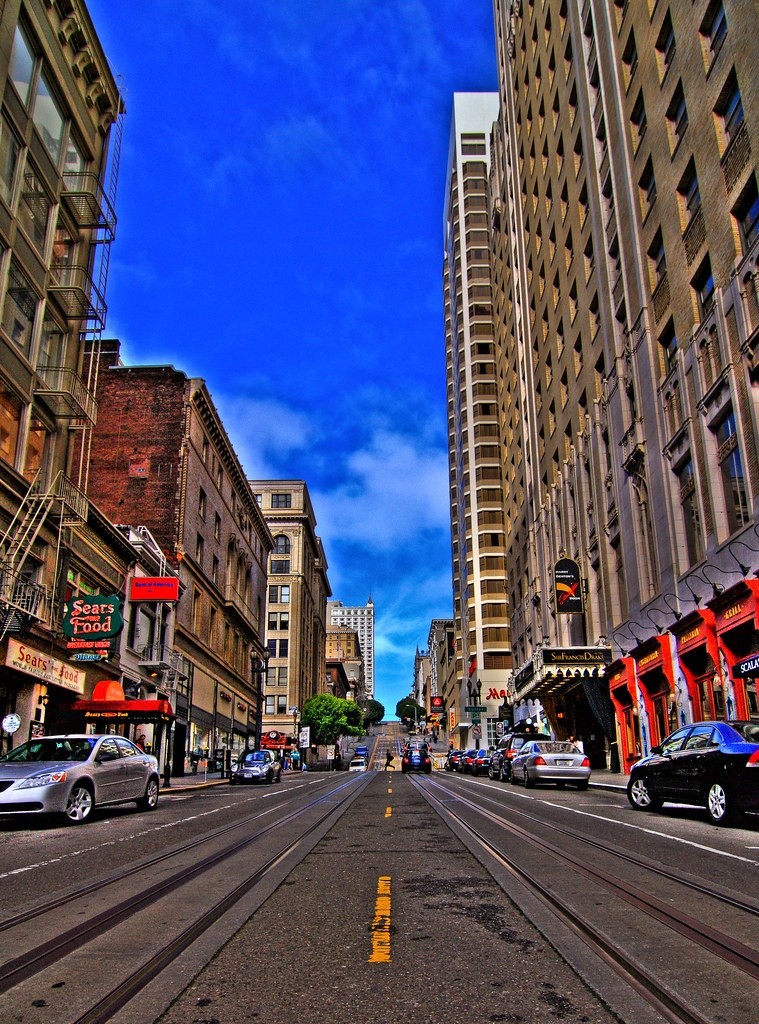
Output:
[0,733,160,825]
[348,756,369,772]
[626,719,759,828]
[445,748,491,777]
[230,746,282,784]
[510,740,592,791]
[400,749,432,774]
[353,745,368,757]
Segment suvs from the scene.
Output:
[488,732,551,781]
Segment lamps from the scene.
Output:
[663,593,694,621]
[702,565,745,596]
[728,541,759,576]
[613,633,634,659]
[685,575,725,607]
[646,608,673,634]
[628,622,658,646]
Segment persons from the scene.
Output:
[335,752,341,770]
[567,736,575,745]
[446,750,452,755]
[385,750,395,771]
[135,735,145,750]
